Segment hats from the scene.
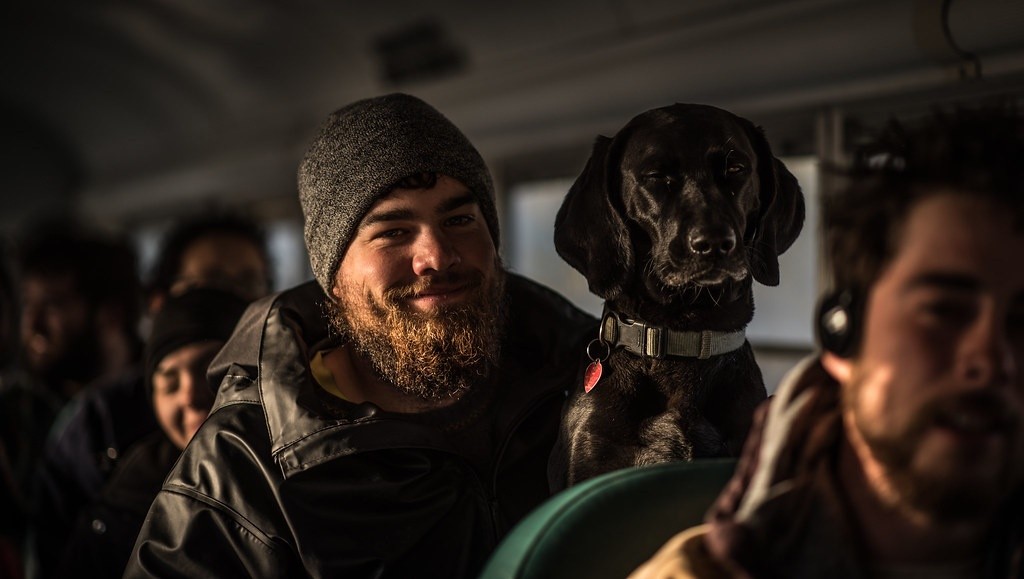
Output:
[298,94,500,304]
[146,285,244,396]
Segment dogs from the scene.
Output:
[552,101,807,494]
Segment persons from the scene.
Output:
[0,209,276,579]
[627,107,1024,579]
[123,94,601,579]
